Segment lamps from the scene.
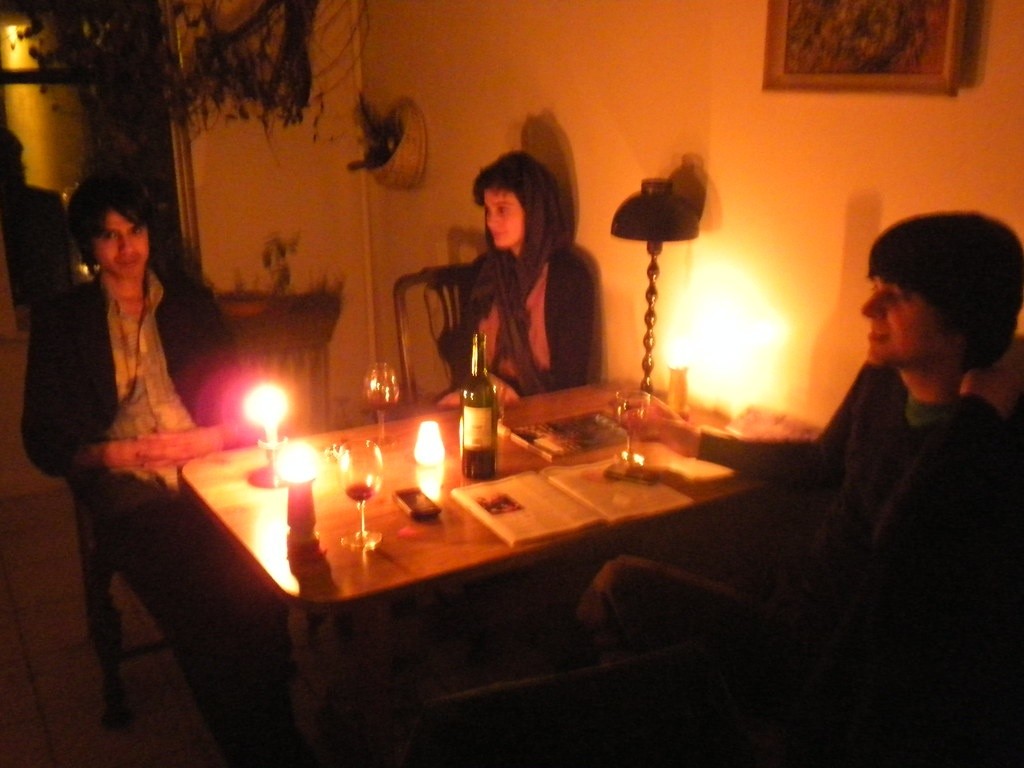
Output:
[611,178,699,391]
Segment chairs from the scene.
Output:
[76,491,175,728]
[392,262,479,418]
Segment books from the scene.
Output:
[507,410,637,461]
[455,442,696,548]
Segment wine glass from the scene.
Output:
[362,361,401,449]
[338,440,383,551]
[616,393,652,473]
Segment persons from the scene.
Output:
[22,173,320,768]
[569,212,1024,768]
[424,151,595,410]
[0,127,72,302]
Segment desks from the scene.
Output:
[182,378,824,768]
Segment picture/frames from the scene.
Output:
[762,0,968,97]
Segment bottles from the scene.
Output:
[458,332,497,481]
